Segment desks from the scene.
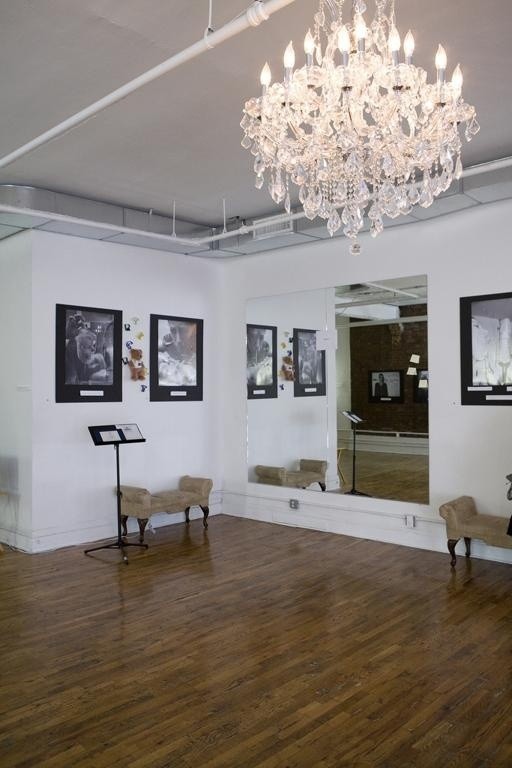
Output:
[335,446,352,489]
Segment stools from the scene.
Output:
[255,459,327,491]
[114,475,213,543]
[438,495,512,567]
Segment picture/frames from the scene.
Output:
[55,303,122,402]
[459,292,512,405]
[293,328,327,397]
[150,313,204,402]
[413,368,428,403]
[368,368,405,404]
[246,324,277,399]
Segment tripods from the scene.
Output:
[84,446,148,565]
[344,424,371,497]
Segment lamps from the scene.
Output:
[240,0,482,256]
[316,288,338,351]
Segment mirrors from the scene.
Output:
[245,274,428,506]
